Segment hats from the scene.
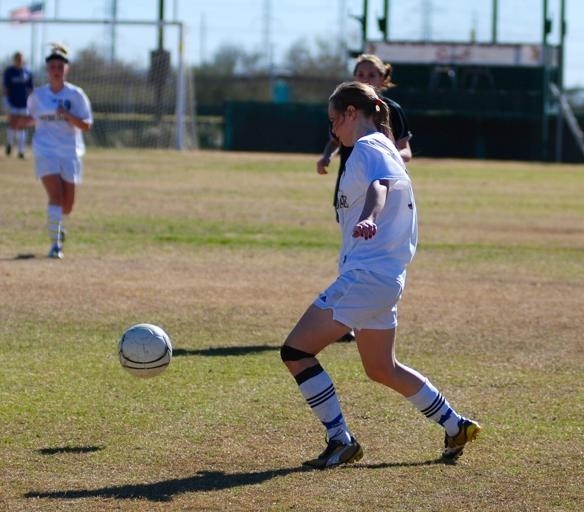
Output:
[45,45,70,64]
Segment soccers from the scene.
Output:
[119,323,172,376]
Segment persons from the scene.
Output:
[14,42,94,259]
[280,81,481,469]
[317,53,413,343]
[2,52,33,159]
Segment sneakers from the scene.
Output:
[442,415,481,463]
[302,433,364,470]
